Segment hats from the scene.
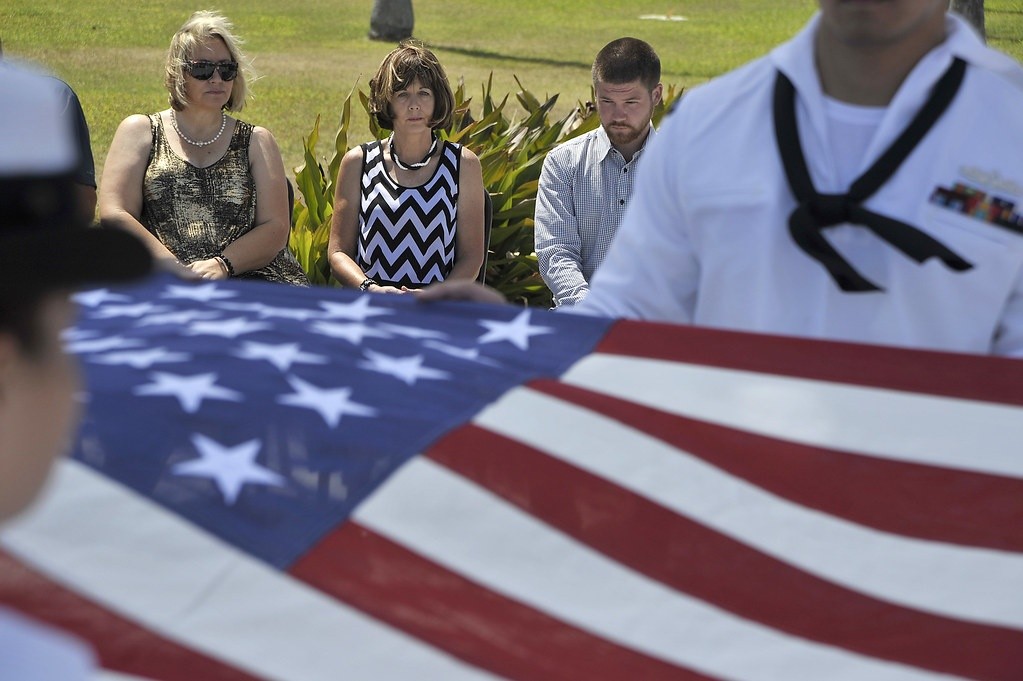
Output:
[0,170,151,327]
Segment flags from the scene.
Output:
[1,275,1023,681]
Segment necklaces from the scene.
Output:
[388,131,438,171]
[171,108,226,146]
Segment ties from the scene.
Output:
[772,58,972,294]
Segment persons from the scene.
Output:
[326,45,485,295]
[534,37,663,311]
[100,12,308,287]
[0,65,98,522]
[576,0,1023,358]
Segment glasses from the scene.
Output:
[184,60,238,81]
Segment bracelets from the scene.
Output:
[212,253,234,279]
[358,279,380,292]
